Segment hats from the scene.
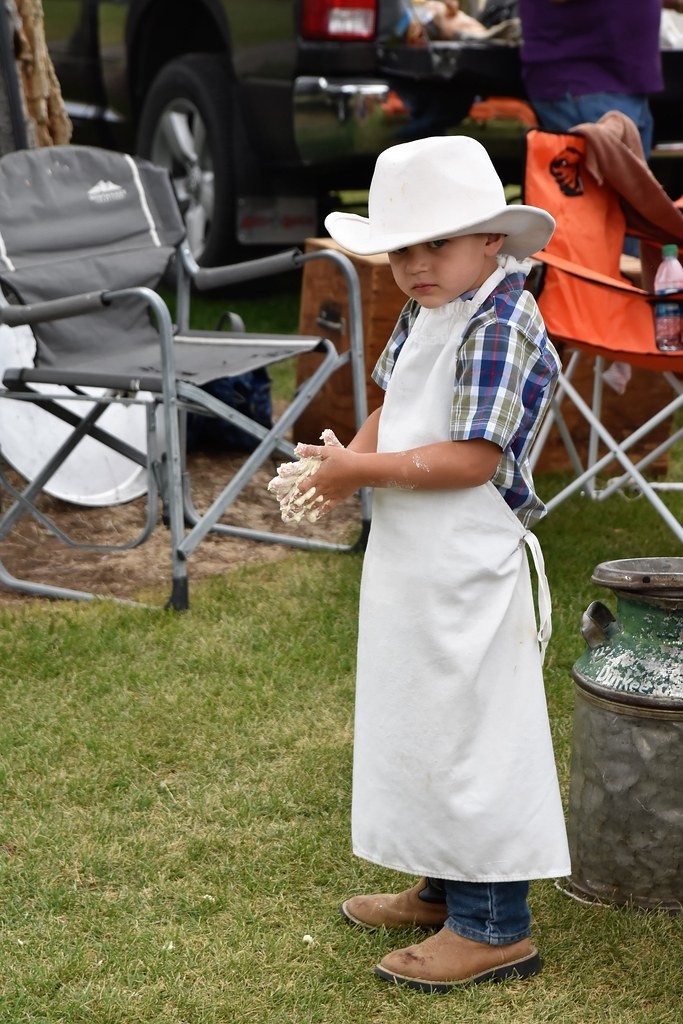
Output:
[325,135,555,260]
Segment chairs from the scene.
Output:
[505,126,683,541]
[1,141,374,612]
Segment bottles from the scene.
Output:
[653,244,683,351]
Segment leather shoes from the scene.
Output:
[340,876,448,927]
[375,927,539,995]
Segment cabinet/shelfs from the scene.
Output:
[294,235,674,476]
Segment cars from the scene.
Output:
[28,1,683,293]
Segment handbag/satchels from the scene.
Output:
[187,312,272,459]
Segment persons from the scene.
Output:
[517,0,683,258]
[262,134,570,993]
